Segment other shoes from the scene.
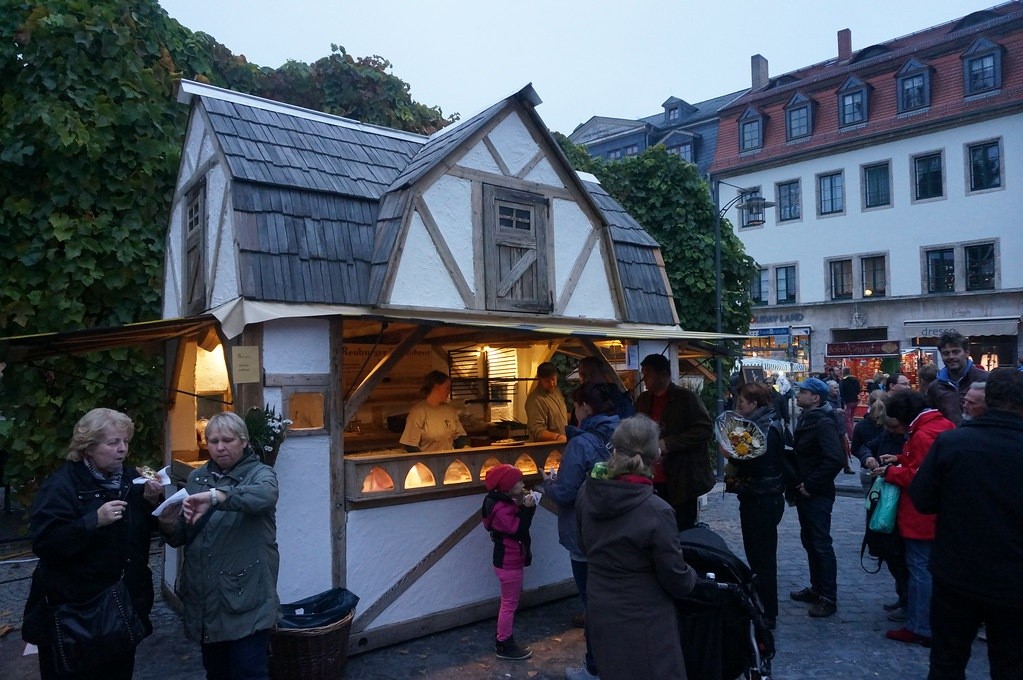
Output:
[565,665,601,680]
[883,598,906,610]
[887,628,931,648]
[844,466,855,474]
[575,612,585,628]
[762,618,777,631]
[890,608,909,622]
[976,625,987,641]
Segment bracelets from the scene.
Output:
[555,434,562,441]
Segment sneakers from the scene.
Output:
[808,597,837,617]
[790,587,820,603]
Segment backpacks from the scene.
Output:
[861,491,900,574]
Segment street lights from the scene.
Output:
[708,174,777,489]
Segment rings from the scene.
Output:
[115,511,119,515]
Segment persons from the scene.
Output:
[538,382,622,680]
[525,362,569,442]
[723,381,787,629]
[786,365,861,618]
[637,354,717,533]
[569,356,633,427]
[575,415,698,680]
[160,412,279,680]
[851,331,1023,680]
[480,463,537,660]
[21,408,167,680]
[398,370,472,453]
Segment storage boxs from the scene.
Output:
[487,423,526,437]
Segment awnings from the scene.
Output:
[340,311,775,430]
[904,316,1022,338]
[737,325,813,339]
[1,316,236,409]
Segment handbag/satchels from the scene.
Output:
[863,471,899,535]
[844,411,854,440]
[50,580,145,675]
[783,426,794,448]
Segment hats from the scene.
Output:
[537,362,557,377]
[486,463,523,491]
[794,378,830,401]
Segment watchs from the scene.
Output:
[209,487,217,505]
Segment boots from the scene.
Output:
[495,633,532,659]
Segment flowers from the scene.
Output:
[728,426,760,456]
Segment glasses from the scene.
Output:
[960,398,976,407]
[896,382,911,387]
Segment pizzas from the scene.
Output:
[136,465,161,483]
